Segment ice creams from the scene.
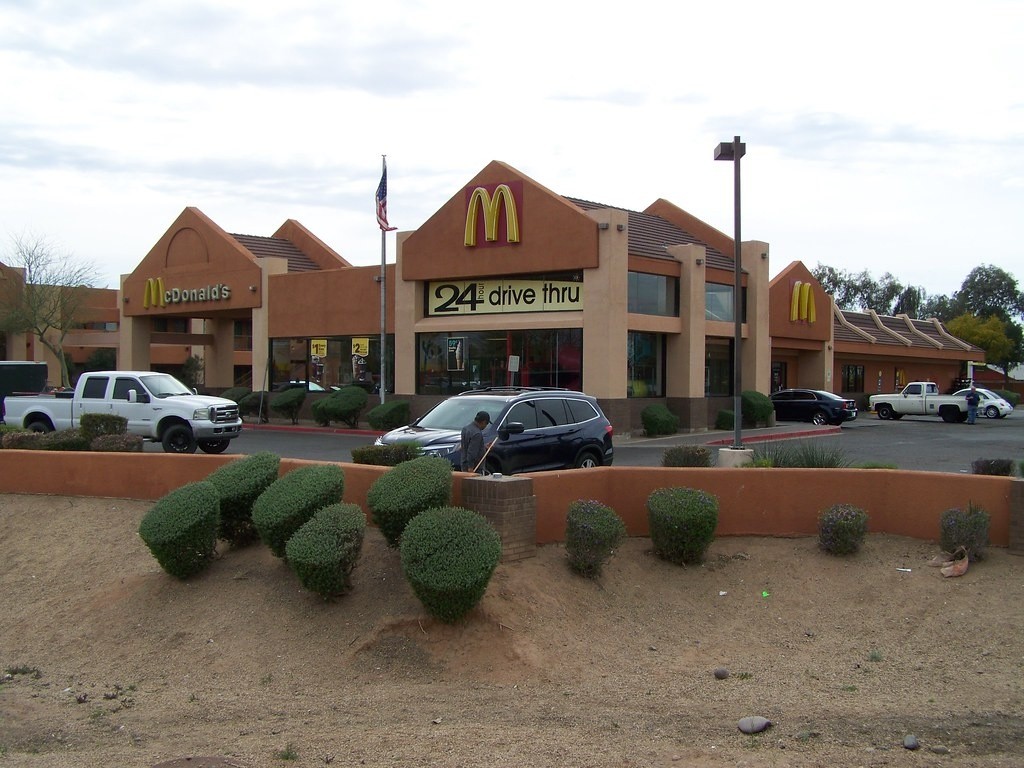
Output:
[455,340,462,369]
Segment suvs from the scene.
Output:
[378,384,614,476]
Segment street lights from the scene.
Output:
[713,137,754,468]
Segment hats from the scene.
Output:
[475,411,493,425]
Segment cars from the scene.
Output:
[770,388,859,426]
[950,387,1013,418]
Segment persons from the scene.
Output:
[964,386,980,425]
[460,411,493,475]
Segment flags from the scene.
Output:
[375,165,398,231]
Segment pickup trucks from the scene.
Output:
[868,381,984,423]
[4,371,243,454]
